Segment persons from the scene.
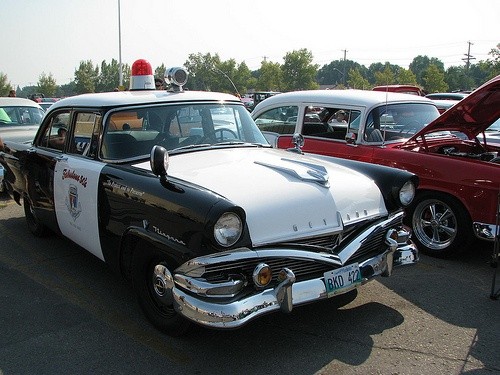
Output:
[329,110,348,126]
[8,90,16,98]
[137,78,174,132]
[54,127,70,144]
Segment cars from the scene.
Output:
[430,99,460,114]
[372,84,422,98]
[241,91,272,114]
[32,93,58,110]
[2,59,419,329]
[0,97,47,193]
[426,92,473,101]
[250,75,500,251]
